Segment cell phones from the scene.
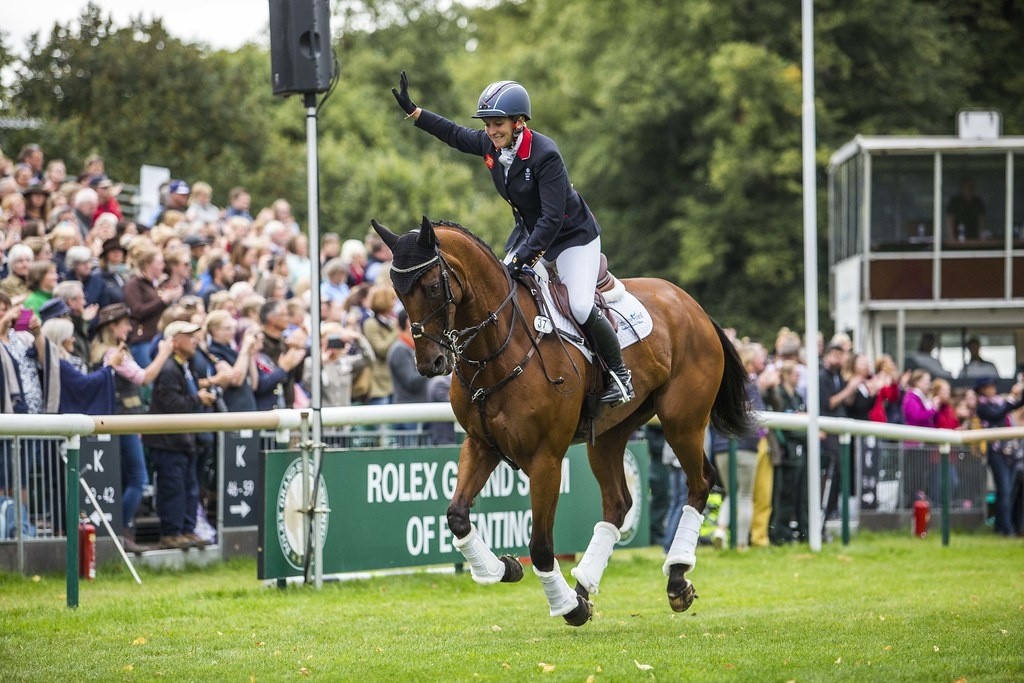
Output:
[328,336,344,348]
[15,309,33,331]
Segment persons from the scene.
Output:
[0,144,1024,547]
[389,68,637,405]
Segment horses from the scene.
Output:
[366,214,744,638]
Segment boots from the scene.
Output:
[578,303,636,404]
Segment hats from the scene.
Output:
[185,236,206,249]
[170,180,190,195]
[39,297,72,322]
[163,320,199,342]
[97,303,130,331]
[98,237,127,260]
[89,174,112,189]
[22,185,50,198]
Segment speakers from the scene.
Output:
[269,0,335,97]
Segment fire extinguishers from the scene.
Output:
[913,489,932,538]
[79,511,96,580]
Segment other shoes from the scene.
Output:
[160,535,195,549]
[185,533,211,546]
[124,538,149,553]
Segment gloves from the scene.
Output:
[392,70,417,118]
[506,255,523,281]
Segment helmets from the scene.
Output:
[471,80,531,121]
